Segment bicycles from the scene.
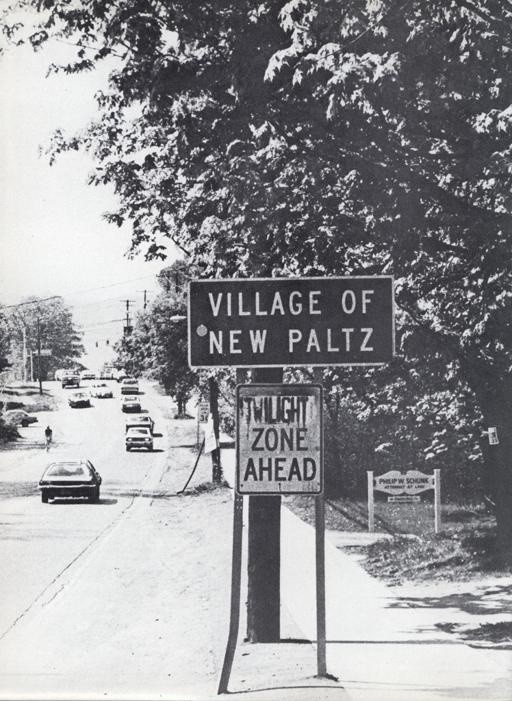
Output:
[45,439,52,451]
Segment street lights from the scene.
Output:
[33,310,45,396]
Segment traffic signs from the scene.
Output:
[233,381,323,497]
[188,273,395,366]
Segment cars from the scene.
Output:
[124,426,154,451]
[54,366,135,388]
[37,458,103,504]
[124,415,155,434]
[120,378,139,394]
[68,391,91,407]
[89,382,114,398]
[121,394,142,412]
[0,408,38,427]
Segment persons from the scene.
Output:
[44,425,53,451]
